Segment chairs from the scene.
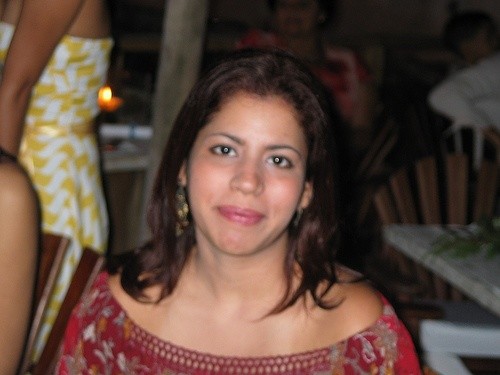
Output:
[355,151,498,310]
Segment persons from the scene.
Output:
[0,0,500,375]
[55,45,423,375]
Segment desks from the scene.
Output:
[383,223,500,318]
